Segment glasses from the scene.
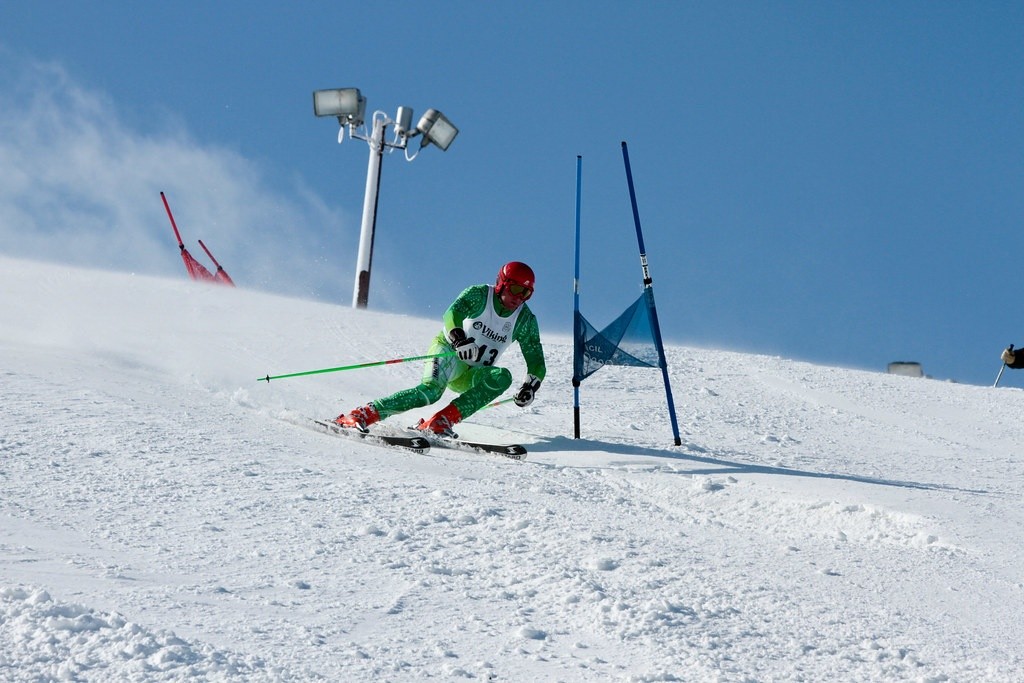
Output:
[503,281,533,302]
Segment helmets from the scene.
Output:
[496,261,534,302]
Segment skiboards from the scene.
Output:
[283,401,528,462]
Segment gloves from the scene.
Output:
[448,327,479,362]
[514,374,541,407]
[1000,348,1015,364]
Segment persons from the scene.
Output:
[1001,347,1024,370]
[332,261,546,440]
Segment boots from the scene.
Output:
[411,402,461,439]
[331,402,380,436]
